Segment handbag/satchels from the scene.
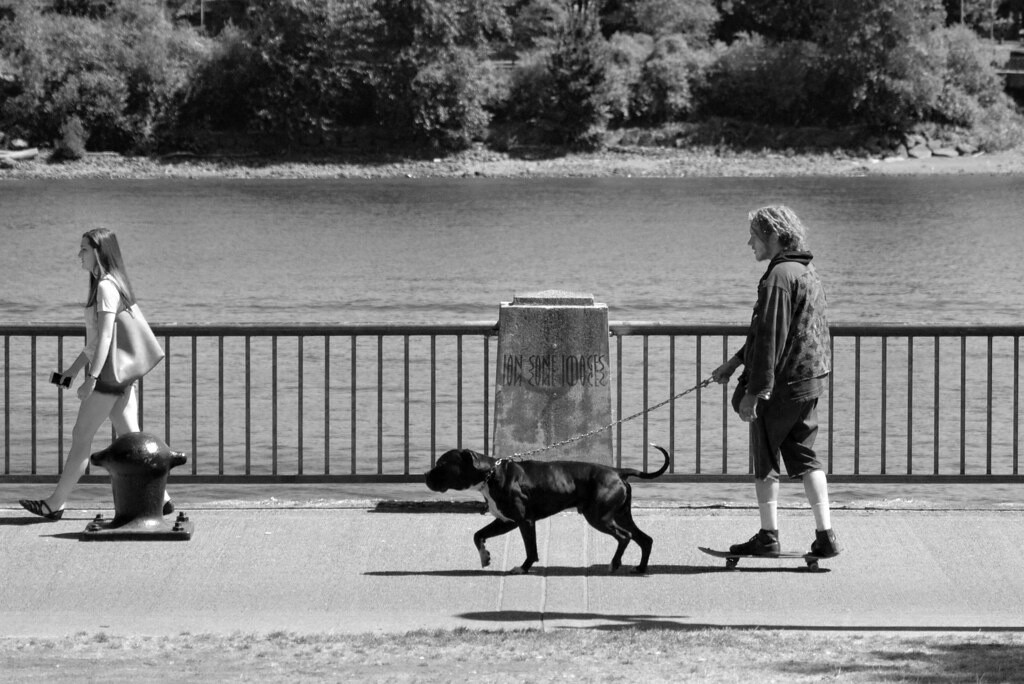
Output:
[83,304,164,387]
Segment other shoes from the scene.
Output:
[19,499,64,521]
[163,499,174,515]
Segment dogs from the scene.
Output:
[423,441,671,576]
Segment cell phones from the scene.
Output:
[49,371,72,389]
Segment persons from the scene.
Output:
[18,229,174,520]
[712,205,837,557]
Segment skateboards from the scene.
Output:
[698,547,847,569]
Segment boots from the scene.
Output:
[730,529,781,555]
[811,529,839,556]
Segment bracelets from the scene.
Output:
[88,373,98,380]
